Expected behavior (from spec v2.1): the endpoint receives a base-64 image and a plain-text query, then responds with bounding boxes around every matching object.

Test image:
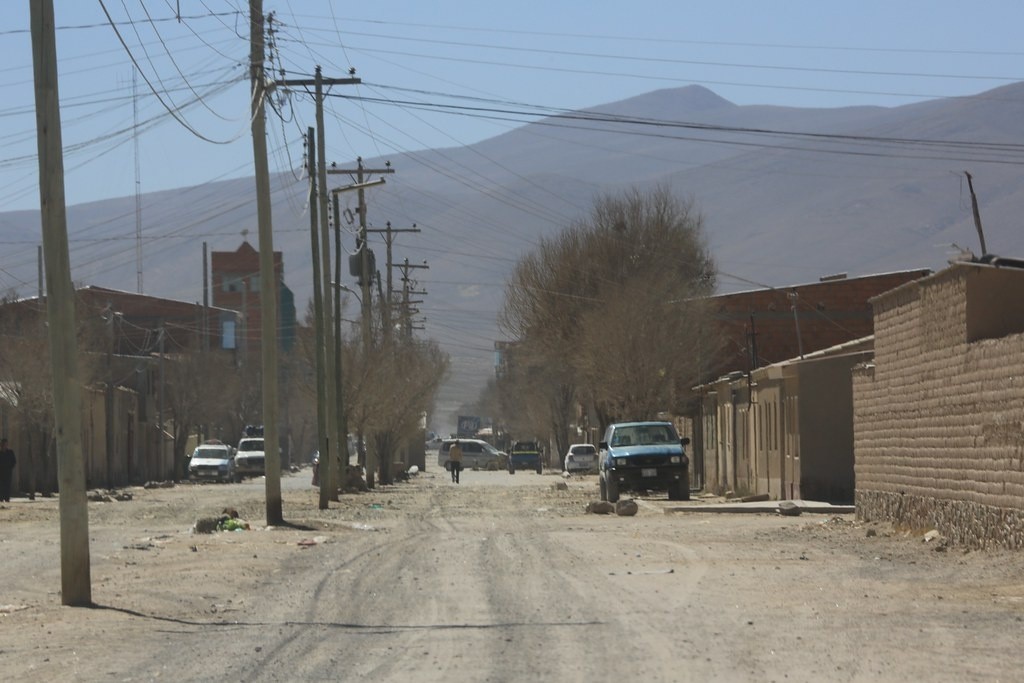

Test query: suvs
[597,422,692,501]
[509,440,544,474]
[236,438,282,478]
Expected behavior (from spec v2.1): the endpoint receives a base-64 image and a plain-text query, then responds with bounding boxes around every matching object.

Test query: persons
[449,439,462,484]
[0,439,17,502]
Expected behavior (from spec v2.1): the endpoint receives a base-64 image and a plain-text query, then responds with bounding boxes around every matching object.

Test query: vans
[438,438,510,470]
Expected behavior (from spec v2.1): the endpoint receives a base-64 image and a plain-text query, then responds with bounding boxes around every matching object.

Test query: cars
[565,444,600,472]
[188,444,235,483]
